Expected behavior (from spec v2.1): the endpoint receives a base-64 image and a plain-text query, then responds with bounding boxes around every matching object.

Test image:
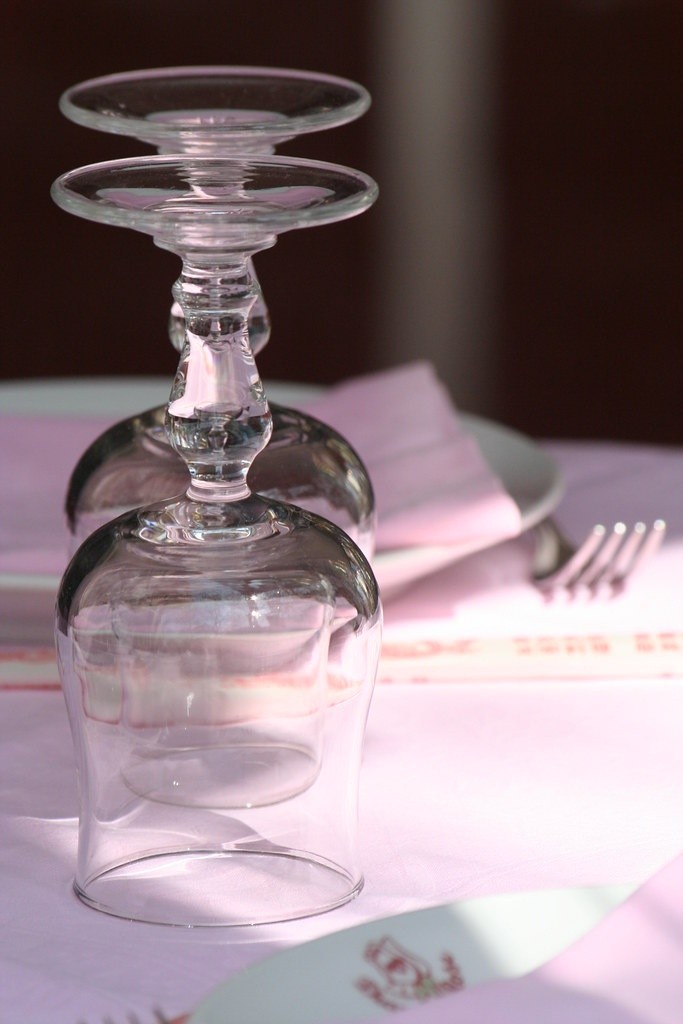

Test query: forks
[528,514,666,606]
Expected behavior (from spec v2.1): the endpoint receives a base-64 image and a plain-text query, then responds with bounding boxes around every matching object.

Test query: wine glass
[61,66,384,807]
[52,151,387,927]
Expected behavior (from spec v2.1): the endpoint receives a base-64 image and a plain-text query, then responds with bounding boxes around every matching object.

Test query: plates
[1,377,567,652]
